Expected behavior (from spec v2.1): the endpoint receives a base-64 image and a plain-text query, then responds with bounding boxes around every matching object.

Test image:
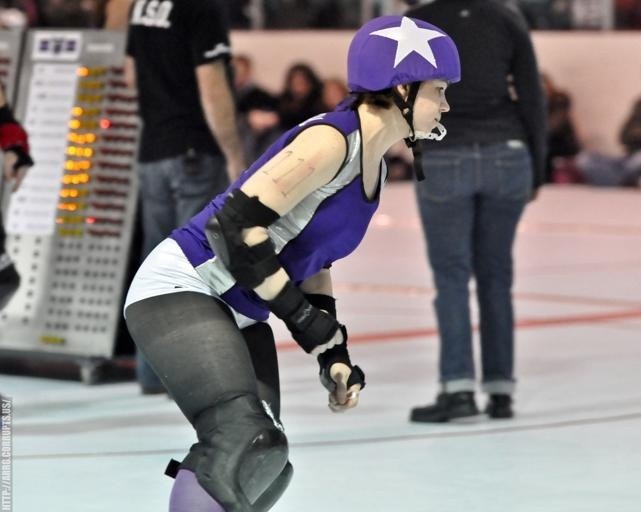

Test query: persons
[111,0,252,394]
[111,13,466,512]
[215,42,641,194]
[392,0,551,423]
[0,81,37,422]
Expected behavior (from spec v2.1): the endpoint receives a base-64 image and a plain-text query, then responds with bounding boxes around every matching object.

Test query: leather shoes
[407,390,482,424]
[484,393,517,421]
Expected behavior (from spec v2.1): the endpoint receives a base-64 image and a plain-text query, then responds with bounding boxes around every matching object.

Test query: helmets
[345,13,464,94]
